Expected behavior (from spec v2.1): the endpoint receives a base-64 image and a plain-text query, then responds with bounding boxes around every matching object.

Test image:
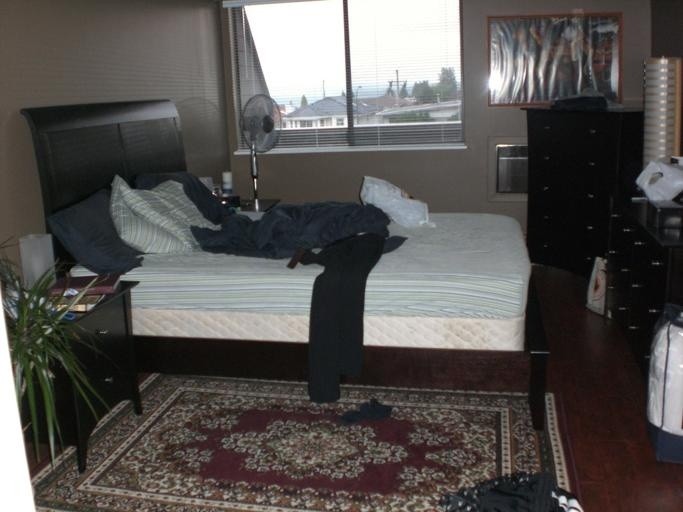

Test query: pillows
[173,172,234,224]
[110,175,191,254]
[124,186,222,248]
[48,189,142,277]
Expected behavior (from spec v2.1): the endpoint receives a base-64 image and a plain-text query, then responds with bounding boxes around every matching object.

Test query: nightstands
[238,199,280,212]
[12,281,142,471]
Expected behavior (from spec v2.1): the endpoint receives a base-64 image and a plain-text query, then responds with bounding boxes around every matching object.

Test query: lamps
[20,231,57,292]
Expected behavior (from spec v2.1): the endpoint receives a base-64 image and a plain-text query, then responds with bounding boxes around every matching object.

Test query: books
[51,274,122,293]
[36,292,104,314]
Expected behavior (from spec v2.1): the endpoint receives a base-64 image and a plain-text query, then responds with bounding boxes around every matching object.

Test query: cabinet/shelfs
[528,110,644,276]
[610,199,683,379]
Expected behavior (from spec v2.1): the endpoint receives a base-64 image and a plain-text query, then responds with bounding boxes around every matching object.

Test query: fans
[240,93,280,211]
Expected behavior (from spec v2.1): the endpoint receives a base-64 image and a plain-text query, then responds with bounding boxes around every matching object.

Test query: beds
[23,100,551,427]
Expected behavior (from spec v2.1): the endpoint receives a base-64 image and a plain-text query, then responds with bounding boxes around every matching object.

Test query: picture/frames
[487,14,621,108]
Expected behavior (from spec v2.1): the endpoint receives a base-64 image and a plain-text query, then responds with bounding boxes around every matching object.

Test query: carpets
[31,374,573,512]
